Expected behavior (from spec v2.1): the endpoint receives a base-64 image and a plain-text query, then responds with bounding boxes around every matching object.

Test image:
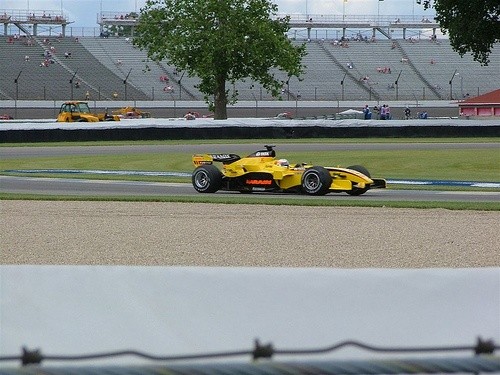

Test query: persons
[374,105,380,119]
[277,158,298,171]
[403,106,411,120]
[0,12,174,101]
[384,104,390,119]
[363,104,370,119]
[380,104,386,120]
[302,16,435,81]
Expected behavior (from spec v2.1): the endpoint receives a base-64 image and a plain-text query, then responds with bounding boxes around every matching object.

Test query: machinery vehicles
[55,101,99,122]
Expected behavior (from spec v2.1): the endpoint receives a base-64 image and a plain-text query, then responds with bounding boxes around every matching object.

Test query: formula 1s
[191,145,387,196]
